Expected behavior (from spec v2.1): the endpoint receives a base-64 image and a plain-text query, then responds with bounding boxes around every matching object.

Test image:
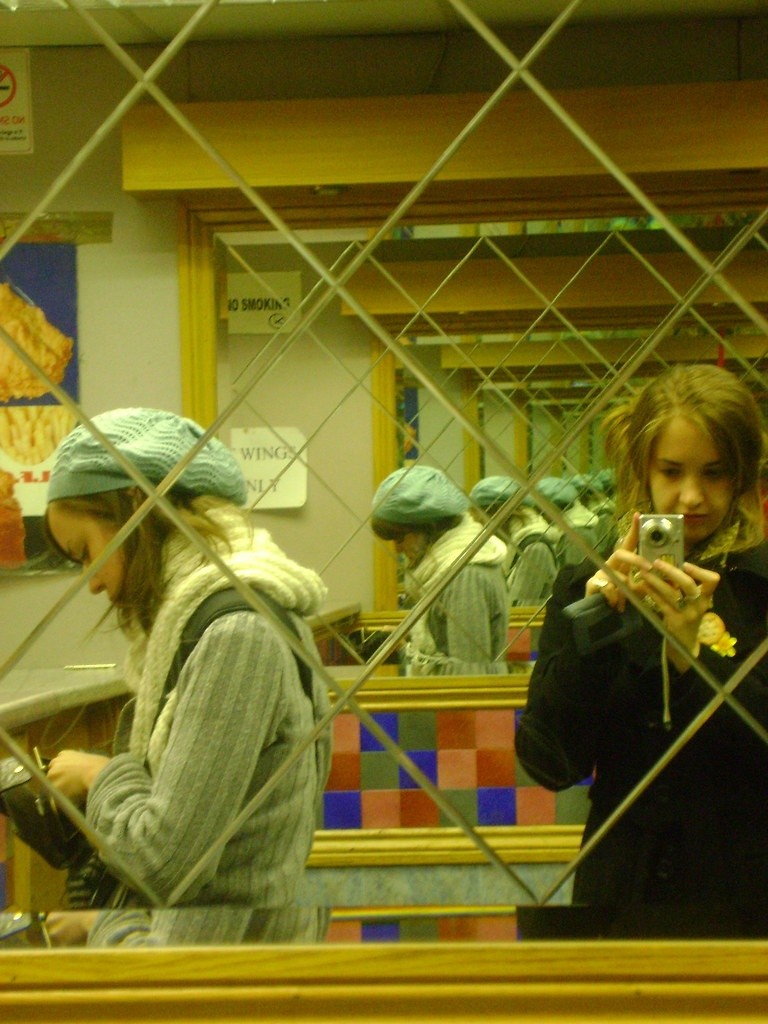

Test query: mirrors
[2,0,767,1017]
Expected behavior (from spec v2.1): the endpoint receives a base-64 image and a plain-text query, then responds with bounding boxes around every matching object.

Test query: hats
[573,471,605,501]
[596,466,621,494]
[470,475,537,509]
[540,477,581,508]
[373,463,468,523]
[46,406,250,505]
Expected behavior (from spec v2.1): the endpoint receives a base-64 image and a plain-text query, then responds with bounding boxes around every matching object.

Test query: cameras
[638,513,686,606]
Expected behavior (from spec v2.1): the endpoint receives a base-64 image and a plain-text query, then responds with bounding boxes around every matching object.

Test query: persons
[519,907,768,942]
[466,476,560,608]
[371,467,511,660]
[569,474,623,553]
[591,468,621,505]
[46,409,335,913]
[402,659,509,677]
[530,478,601,571]
[513,364,767,904]
[39,911,331,952]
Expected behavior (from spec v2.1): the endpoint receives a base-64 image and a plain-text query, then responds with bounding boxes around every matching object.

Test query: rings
[597,580,613,596]
[674,597,686,610]
[687,589,702,603]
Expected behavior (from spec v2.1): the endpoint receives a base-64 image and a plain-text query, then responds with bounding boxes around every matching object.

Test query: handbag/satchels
[1,755,88,870]
